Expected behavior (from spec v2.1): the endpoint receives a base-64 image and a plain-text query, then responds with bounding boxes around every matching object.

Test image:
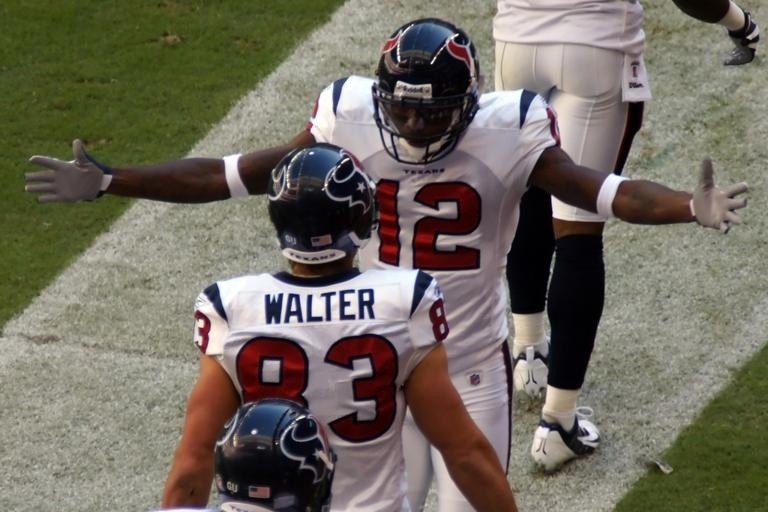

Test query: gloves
[24,139,111,204]
[724,12,759,65]
[690,157,748,232]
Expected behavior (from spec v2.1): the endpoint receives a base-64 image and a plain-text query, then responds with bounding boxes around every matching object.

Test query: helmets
[371,18,480,165]
[268,143,376,266]
[215,398,335,512]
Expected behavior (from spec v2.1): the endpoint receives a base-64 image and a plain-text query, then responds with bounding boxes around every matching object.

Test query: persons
[163,396,337,512]
[492,0,759,472]
[24,19,748,512]
[161,145,520,512]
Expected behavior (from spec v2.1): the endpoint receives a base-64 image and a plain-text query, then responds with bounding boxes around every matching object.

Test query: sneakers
[530,419,600,472]
[514,338,549,396]
